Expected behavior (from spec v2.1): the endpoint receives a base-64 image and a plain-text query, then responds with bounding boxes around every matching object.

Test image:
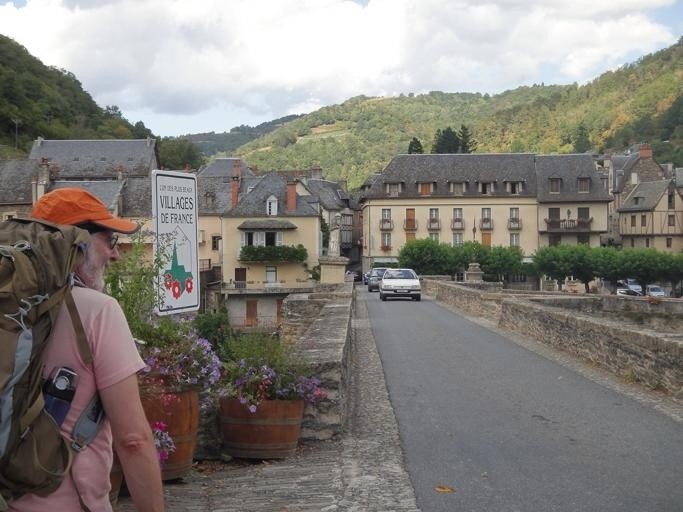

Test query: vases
[140,385,218,481]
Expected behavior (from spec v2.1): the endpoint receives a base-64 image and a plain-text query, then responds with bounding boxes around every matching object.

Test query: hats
[31,187,139,234]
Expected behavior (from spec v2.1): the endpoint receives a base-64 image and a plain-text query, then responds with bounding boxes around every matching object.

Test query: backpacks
[1,214,104,512]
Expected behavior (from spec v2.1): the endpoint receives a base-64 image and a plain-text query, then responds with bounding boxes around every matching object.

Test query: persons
[7,188,166,512]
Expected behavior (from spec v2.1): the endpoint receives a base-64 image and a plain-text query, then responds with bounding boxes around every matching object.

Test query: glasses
[107,234,117,249]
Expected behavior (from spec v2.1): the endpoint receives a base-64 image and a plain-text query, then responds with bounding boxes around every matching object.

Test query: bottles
[41,364,79,427]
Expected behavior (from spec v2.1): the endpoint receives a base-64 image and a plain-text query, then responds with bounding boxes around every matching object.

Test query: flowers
[126,318,329,461]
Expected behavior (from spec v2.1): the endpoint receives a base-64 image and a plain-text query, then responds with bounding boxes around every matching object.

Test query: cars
[364,267,421,301]
[616,279,667,297]
